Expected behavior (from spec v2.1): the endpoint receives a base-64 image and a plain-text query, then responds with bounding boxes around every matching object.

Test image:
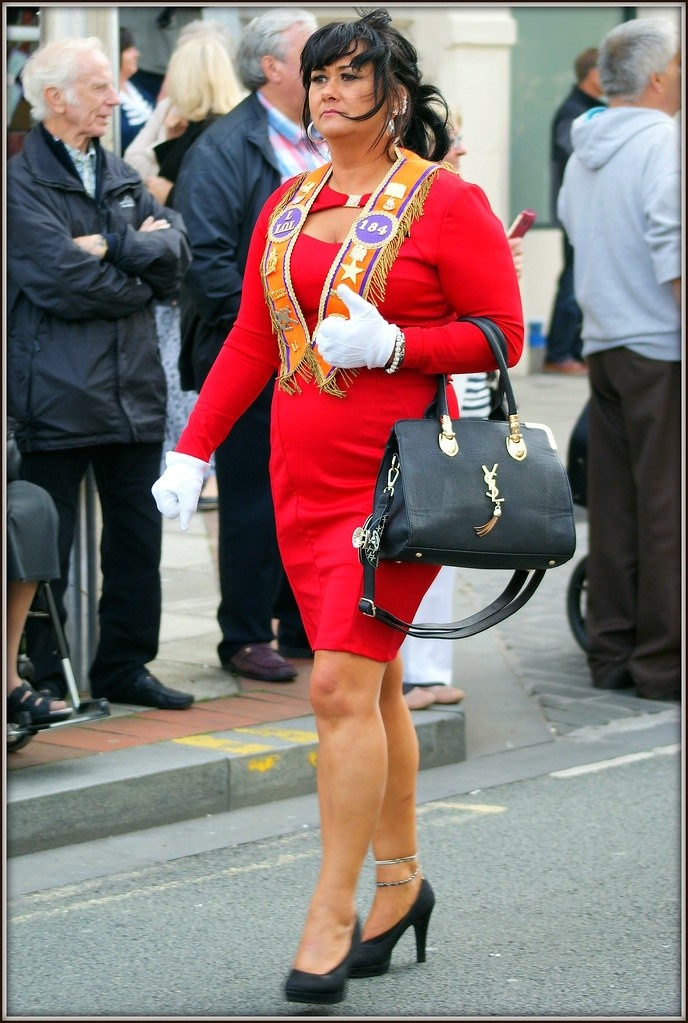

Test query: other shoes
[198,492,222,511]
[539,356,592,375]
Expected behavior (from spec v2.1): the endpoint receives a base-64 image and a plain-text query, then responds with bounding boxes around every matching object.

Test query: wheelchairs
[7,574,110,753]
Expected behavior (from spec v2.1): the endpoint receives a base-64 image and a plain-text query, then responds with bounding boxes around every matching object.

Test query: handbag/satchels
[350,317,576,639]
[567,397,588,509]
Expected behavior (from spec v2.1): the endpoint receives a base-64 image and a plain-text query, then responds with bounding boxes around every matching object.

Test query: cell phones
[505,209,536,240]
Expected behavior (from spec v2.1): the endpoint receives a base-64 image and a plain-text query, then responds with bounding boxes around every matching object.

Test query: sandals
[8,679,74,720]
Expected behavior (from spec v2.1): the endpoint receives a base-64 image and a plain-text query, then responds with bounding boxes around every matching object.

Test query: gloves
[151,451,211,531]
[316,284,395,369]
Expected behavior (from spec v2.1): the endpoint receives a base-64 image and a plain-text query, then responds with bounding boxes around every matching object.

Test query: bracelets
[385,327,405,375]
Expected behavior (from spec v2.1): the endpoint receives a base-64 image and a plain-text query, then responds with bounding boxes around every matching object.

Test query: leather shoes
[92,673,194,710]
[221,642,298,680]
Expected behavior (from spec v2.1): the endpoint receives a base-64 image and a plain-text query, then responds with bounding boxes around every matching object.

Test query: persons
[424,104,525,420]
[6,416,74,718]
[171,9,332,682]
[151,9,525,1006]
[555,17,681,700]
[6,36,193,710]
[126,20,245,509]
[119,24,157,158]
[541,47,608,378]
[397,566,465,711]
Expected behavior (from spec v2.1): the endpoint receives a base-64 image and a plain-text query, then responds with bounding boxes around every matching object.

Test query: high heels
[284,915,361,1003]
[348,878,436,977]
